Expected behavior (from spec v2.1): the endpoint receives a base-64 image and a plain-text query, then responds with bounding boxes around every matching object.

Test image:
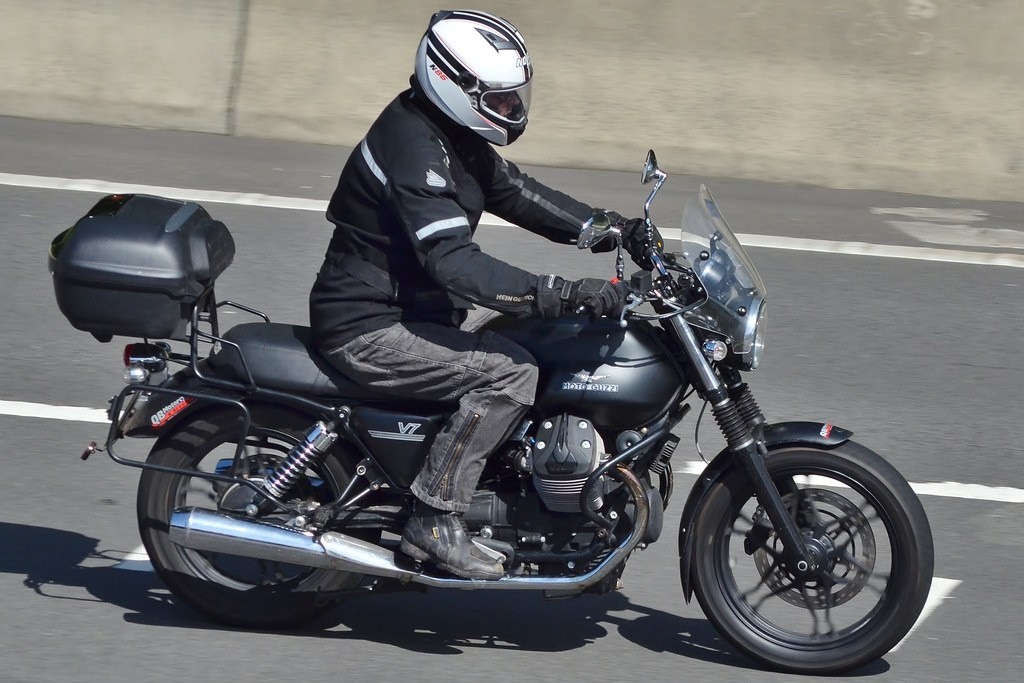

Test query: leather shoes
[399,497,504,581]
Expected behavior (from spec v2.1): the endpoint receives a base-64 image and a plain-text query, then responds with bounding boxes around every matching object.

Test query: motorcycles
[47,148,936,677]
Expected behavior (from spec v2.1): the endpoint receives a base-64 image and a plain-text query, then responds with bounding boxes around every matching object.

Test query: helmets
[414,9,533,146]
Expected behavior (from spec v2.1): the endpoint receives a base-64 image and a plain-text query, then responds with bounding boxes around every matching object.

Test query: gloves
[589,211,664,272]
[537,274,622,324]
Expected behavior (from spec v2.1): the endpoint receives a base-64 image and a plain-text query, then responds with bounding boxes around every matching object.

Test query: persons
[308,9,664,581]
